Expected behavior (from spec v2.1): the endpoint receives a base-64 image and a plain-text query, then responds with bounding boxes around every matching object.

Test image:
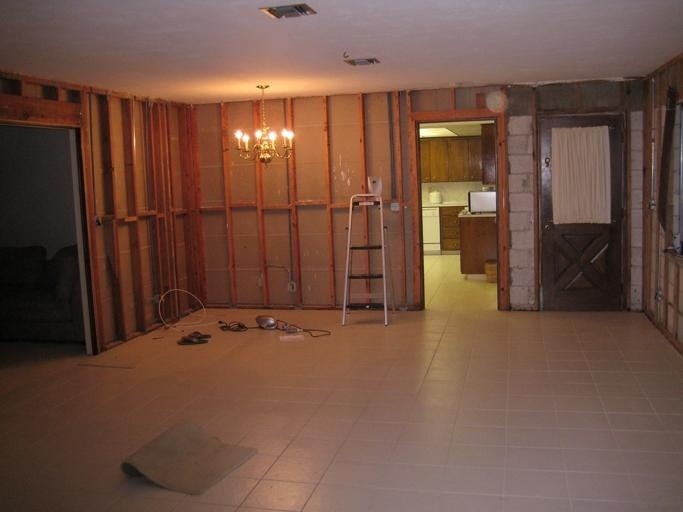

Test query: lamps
[234,84,294,167]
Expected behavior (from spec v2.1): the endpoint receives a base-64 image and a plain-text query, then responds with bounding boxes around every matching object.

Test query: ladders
[341,193,388,325]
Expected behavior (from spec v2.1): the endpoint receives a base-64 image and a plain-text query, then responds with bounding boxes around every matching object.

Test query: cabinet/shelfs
[419,135,482,183]
[438,207,467,255]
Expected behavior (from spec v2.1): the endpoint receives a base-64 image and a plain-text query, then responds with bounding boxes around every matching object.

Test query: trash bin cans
[485,260,498,282]
